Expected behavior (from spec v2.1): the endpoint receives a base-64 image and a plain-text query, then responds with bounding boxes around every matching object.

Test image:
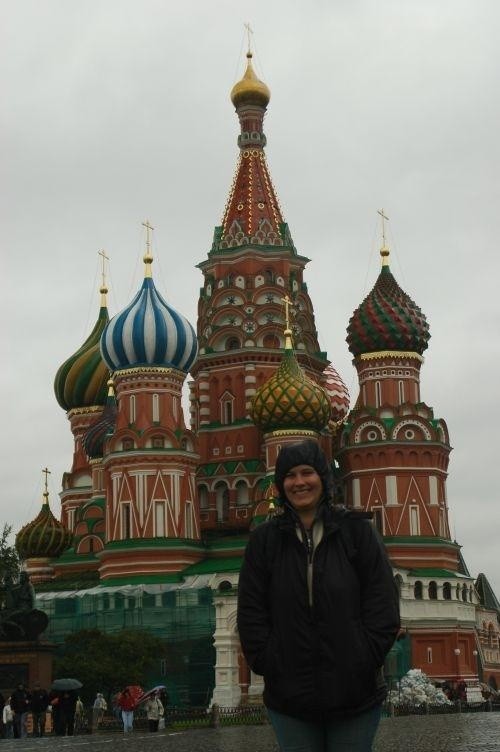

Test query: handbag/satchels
[159,717,165,730]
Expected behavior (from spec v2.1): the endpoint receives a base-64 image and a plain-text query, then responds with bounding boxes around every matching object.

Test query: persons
[1,677,109,740]
[236,435,402,751]
[117,688,136,735]
[143,692,165,732]
